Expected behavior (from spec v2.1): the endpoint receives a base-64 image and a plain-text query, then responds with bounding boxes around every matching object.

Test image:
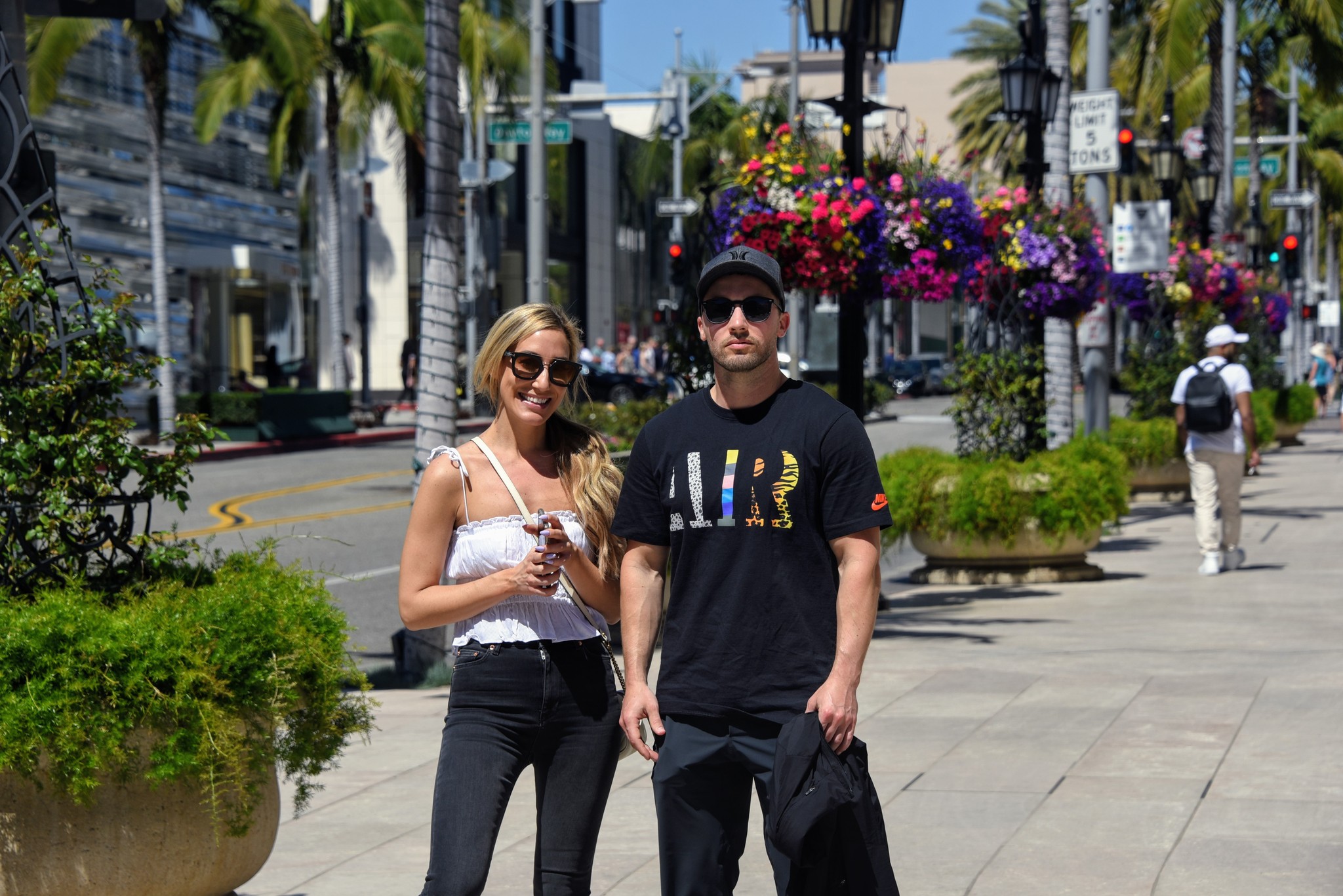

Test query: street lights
[1190,157,1221,250]
[1147,117,1184,219]
[802,0,905,424]
[996,22,1064,461]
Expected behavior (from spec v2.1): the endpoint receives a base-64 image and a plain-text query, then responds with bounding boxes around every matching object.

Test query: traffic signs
[1267,189,1320,210]
[655,196,702,217]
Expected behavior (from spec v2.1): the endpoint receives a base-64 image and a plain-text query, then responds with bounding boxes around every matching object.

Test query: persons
[1170,324,1260,575]
[399,331,420,402]
[577,336,664,381]
[399,302,625,896]
[611,246,899,896]
[1305,341,1343,430]
[334,331,352,391]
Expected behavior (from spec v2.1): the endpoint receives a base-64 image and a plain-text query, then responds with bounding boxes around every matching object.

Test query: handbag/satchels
[615,691,647,760]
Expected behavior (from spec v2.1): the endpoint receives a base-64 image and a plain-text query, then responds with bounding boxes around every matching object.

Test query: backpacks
[1184,362,1237,433]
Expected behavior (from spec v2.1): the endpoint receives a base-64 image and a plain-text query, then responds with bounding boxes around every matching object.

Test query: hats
[697,245,786,313]
[1309,341,1327,359]
[1205,324,1249,349]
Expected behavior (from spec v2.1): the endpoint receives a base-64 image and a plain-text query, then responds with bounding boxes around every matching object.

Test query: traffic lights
[1117,125,1136,176]
[1302,304,1317,319]
[1283,233,1300,279]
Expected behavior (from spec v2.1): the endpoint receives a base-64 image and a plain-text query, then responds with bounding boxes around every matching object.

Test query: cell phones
[537,508,553,590]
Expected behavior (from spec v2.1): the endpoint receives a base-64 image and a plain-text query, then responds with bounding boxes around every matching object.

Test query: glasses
[701,296,782,324]
[502,351,582,387]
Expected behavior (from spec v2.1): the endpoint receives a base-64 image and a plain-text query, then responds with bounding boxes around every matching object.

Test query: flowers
[711,108,1289,338]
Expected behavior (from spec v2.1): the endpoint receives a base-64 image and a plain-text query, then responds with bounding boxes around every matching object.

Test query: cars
[566,361,667,411]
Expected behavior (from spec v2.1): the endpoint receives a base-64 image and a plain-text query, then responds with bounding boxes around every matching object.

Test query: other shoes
[1222,548,1245,570]
[1197,556,1219,575]
